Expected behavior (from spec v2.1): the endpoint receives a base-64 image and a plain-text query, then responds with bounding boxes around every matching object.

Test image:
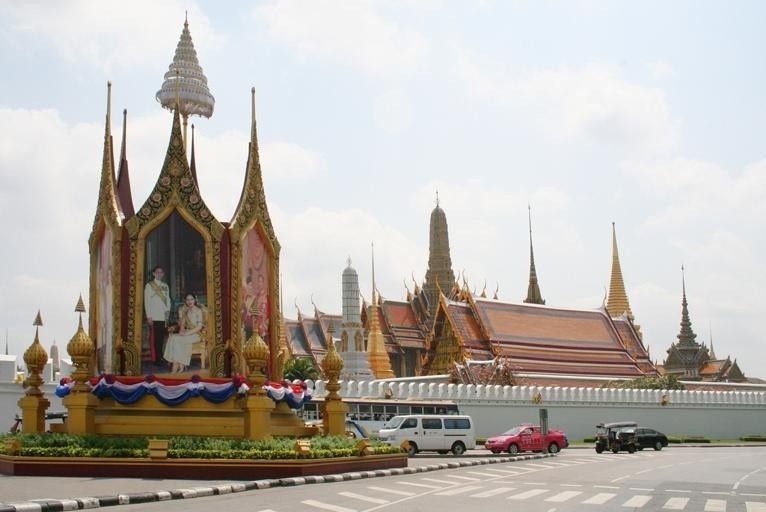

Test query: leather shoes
[157,360,188,375]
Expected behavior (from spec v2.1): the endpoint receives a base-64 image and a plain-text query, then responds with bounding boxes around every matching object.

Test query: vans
[378,415,476,457]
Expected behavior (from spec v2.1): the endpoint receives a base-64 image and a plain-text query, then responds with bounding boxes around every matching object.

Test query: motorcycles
[595,422,638,454]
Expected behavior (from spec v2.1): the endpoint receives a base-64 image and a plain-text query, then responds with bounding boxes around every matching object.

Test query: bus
[297,397,461,439]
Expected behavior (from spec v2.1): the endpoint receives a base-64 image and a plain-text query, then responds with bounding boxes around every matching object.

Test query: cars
[486,424,569,454]
[634,428,669,451]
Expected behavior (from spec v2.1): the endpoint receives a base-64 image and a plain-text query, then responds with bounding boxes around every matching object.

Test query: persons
[243,274,255,340]
[96,264,106,375]
[144,266,173,366]
[255,273,268,340]
[103,263,112,373]
[161,290,203,375]
